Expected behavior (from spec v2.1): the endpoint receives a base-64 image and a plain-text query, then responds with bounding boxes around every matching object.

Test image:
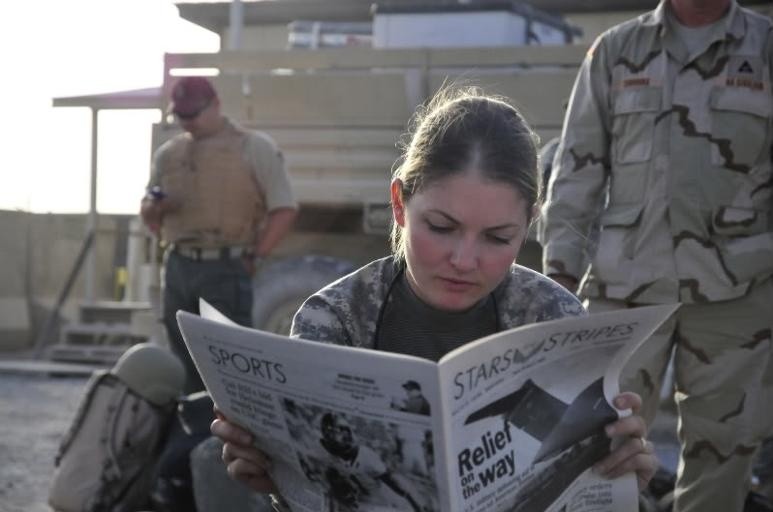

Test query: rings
[638,436,649,451]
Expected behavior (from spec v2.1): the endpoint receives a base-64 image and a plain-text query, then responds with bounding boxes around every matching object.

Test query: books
[174,297,683,512]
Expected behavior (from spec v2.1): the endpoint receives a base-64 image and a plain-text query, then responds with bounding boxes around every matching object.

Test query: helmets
[110,342,186,407]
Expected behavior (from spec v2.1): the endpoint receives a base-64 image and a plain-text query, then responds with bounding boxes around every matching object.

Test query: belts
[178,247,242,260]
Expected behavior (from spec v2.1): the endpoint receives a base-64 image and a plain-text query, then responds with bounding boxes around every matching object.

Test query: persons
[537,98,611,283]
[137,79,298,394]
[209,95,660,508]
[535,1,773,510]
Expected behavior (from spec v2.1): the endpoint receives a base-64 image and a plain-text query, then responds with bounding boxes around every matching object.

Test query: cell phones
[150,185,167,201]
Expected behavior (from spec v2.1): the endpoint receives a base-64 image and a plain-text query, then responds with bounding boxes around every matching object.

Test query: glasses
[177,101,209,118]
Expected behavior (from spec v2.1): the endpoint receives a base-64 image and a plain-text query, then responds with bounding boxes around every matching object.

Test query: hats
[165,76,213,115]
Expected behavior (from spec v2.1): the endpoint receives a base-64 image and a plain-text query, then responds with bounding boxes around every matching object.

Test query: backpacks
[47,368,177,512]
